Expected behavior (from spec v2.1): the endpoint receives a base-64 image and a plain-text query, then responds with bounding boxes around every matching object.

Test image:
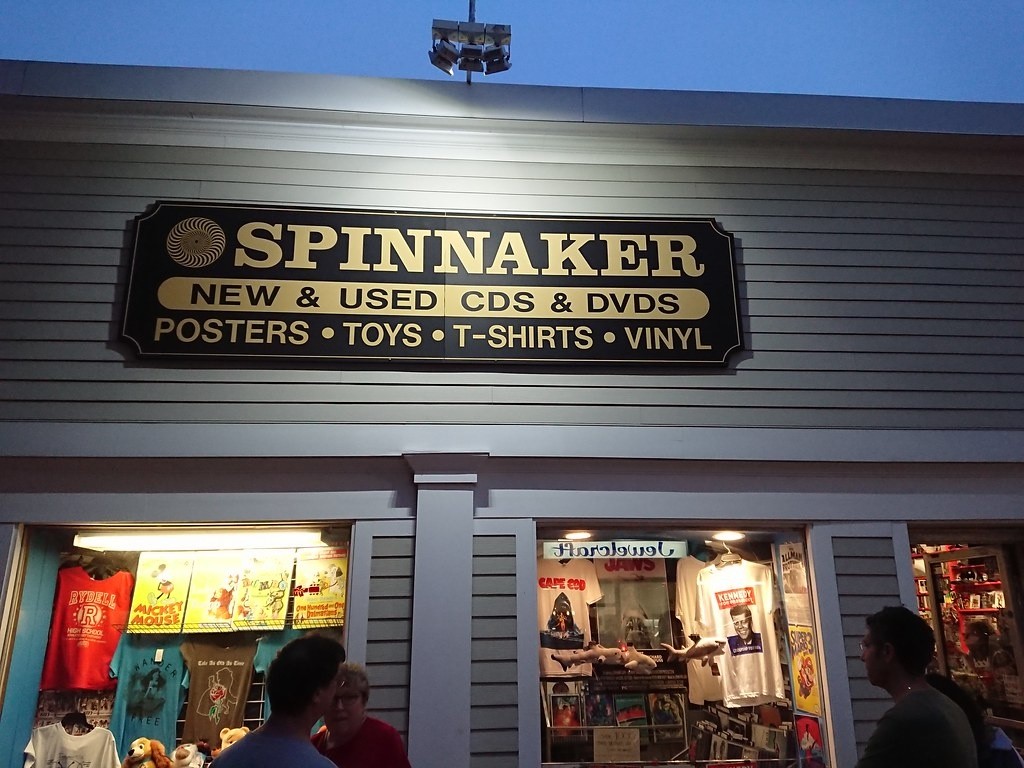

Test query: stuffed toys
[946,616,1018,702]
[550,633,726,675]
[121,724,250,768]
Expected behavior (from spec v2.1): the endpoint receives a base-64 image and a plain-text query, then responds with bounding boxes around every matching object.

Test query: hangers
[79,553,119,580]
[693,541,741,572]
[61,696,97,735]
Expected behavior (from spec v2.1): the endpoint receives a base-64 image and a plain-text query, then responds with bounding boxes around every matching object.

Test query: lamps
[74,528,332,553]
[427,0,518,84]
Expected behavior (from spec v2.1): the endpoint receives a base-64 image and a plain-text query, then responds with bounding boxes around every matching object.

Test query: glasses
[859,641,884,651]
[963,632,973,640]
[328,693,366,708]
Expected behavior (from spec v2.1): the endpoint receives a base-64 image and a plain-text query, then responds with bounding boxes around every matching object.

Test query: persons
[726,605,763,655]
[207,634,346,768]
[853,606,1024,768]
[800,722,823,764]
[310,661,413,768]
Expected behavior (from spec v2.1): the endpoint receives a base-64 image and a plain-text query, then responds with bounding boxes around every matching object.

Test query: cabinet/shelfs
[911,544,1022,701]
